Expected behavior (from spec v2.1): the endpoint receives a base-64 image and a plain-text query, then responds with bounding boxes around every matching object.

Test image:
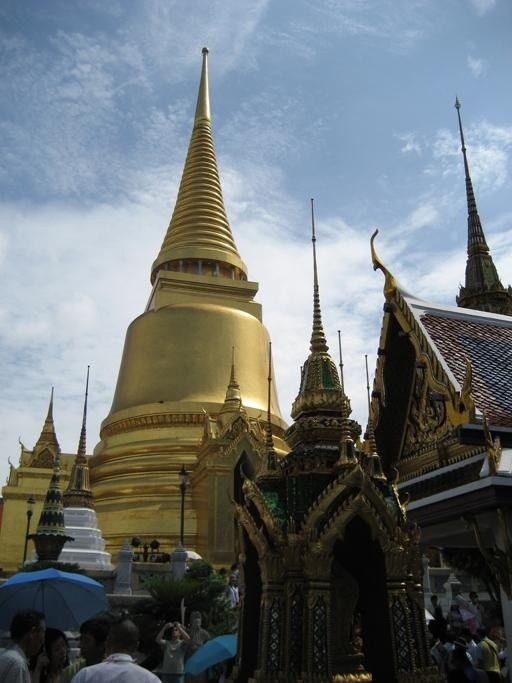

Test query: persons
[218,561,242,611]
[187,611,213,656]
[1,606,47,683]
[425,589,510,683]
[155,620,192,683]
[28,626,71,682]
[69,616,162,682]
[54,614,109,682]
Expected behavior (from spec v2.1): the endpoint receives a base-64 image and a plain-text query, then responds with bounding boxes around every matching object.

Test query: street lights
[22,492,38,562]
[177,461,191,547]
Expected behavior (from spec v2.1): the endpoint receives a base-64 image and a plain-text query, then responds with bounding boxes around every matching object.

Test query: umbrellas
[0,566,109,632]
[184,633,237,675]
